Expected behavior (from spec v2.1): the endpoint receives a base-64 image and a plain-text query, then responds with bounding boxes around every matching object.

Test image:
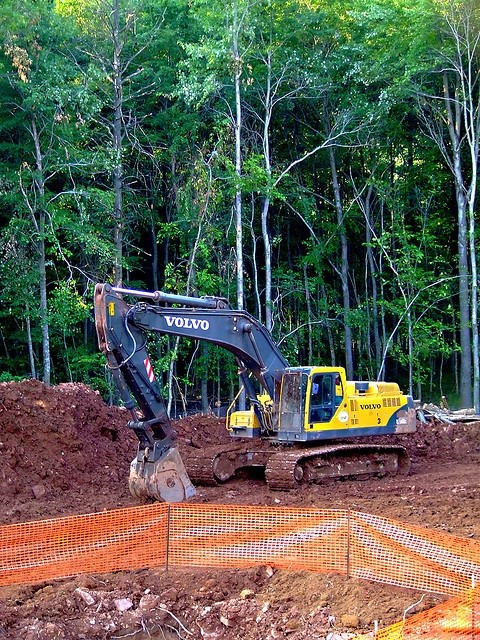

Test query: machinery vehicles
[82,277,420,507]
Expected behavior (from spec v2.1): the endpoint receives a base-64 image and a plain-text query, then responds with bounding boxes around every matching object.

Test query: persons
[312,375,327,405]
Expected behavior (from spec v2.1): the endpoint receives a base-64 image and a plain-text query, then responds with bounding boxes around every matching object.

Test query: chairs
[309,374,324,410]
